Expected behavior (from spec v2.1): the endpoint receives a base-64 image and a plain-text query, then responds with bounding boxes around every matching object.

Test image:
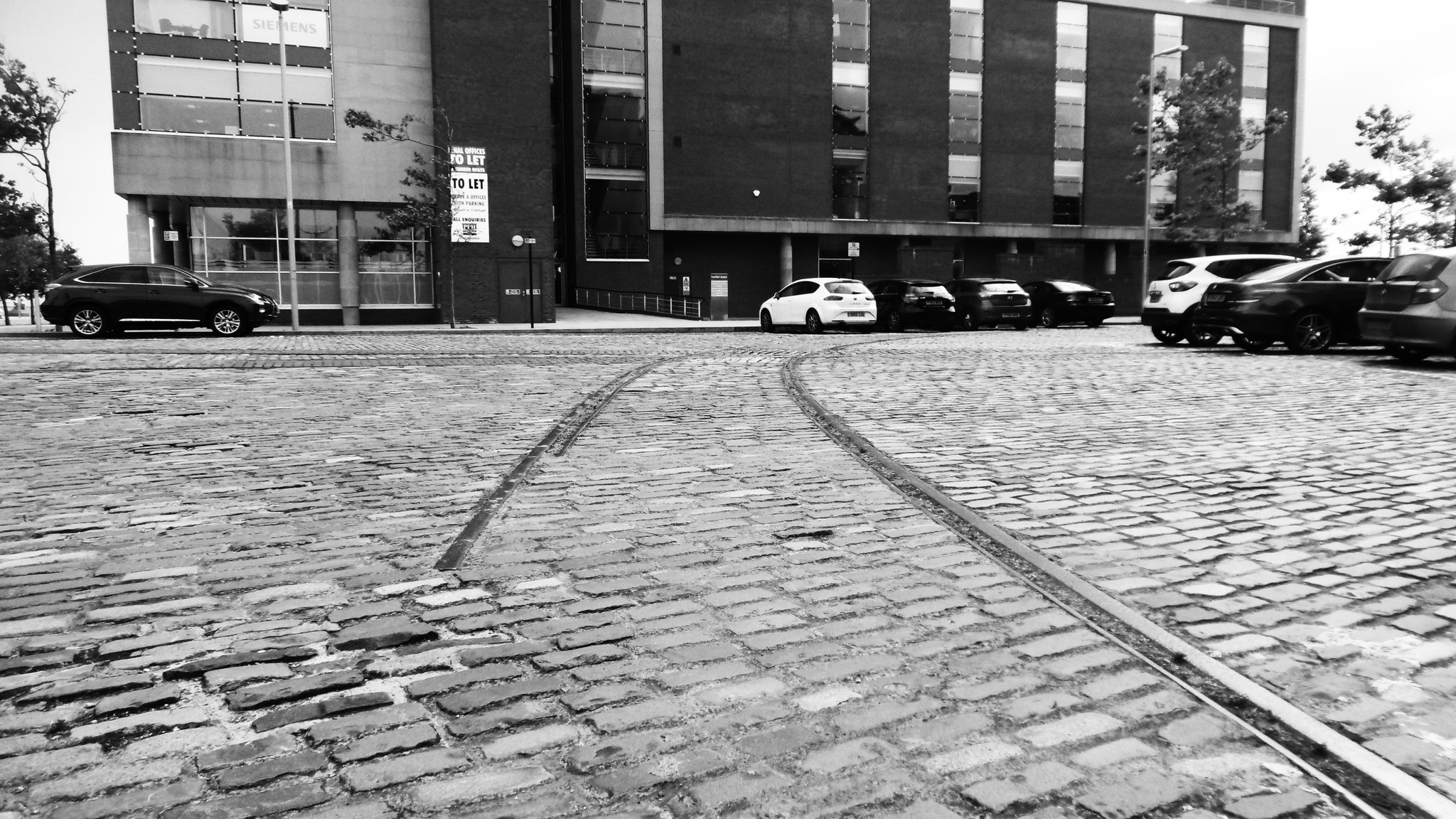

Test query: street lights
[1140,45,1189,321]
[270,0,301,330]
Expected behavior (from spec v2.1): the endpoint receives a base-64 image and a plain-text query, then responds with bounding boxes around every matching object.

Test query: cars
[1358,246,1456,371]
[1195,256,1400,354]
[759,278,877,334]
[944,277,1032,330]
[40,263,279,336]
[865,279,956,332]
[1020,279,1116,329]
[1142,254,1302,348]
